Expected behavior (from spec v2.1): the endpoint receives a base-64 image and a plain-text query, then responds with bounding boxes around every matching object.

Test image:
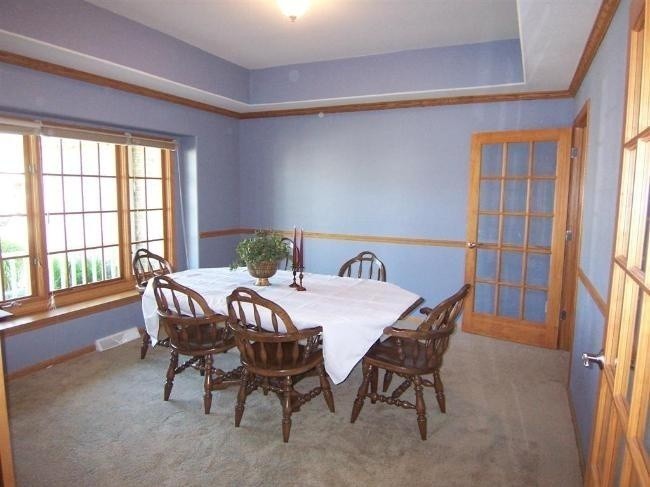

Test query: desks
[141,266,425,388]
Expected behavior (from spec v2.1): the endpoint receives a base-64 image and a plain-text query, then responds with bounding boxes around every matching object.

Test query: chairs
[133,250,205,376]
[225,288,335,442]
[279,238,299,271]
[337,251,386,282]
[350,284,472,440]
[153,276,241,415]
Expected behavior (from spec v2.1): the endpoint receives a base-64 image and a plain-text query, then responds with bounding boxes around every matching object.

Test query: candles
[293,227,297,264]
[299,228,303,267]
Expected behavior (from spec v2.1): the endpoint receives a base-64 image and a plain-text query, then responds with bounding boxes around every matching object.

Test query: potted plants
[230,230,292,285]
